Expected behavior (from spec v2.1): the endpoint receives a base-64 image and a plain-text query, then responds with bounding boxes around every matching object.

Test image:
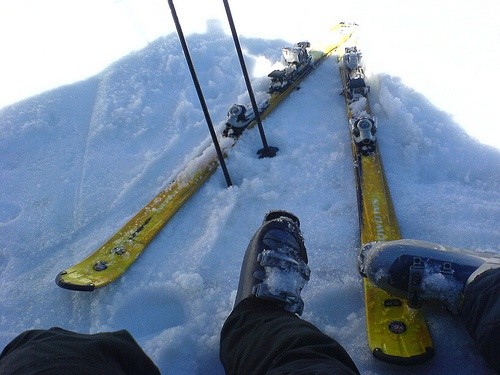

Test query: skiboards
[54,22,437,366]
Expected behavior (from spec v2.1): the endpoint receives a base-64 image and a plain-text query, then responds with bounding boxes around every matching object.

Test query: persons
[0,210,361,375]
[356,239,500,375]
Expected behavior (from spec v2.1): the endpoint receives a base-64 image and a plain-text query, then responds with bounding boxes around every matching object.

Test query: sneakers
[235,218,311,317]
[367,239,500,316]
